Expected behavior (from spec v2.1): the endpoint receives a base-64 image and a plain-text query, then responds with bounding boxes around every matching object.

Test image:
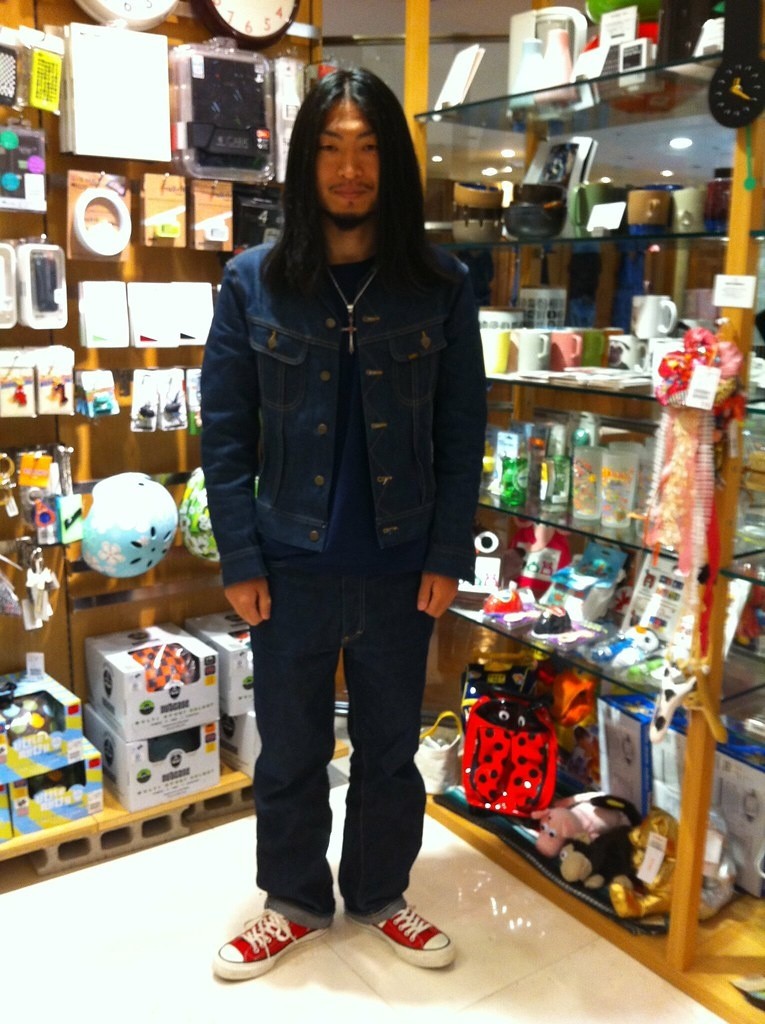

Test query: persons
[200,66,488,982]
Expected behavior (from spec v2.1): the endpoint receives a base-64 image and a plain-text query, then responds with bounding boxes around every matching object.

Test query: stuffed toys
[531,796,737,917]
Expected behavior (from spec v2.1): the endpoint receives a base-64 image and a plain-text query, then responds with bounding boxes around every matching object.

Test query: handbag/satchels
[412,710,464,795]
[462,669,559,818]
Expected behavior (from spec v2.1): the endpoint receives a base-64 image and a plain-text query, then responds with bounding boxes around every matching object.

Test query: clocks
[191,0,300,51]
[74,0,179,30]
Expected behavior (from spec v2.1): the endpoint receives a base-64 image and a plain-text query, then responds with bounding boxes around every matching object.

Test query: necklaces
[329,269,375,354]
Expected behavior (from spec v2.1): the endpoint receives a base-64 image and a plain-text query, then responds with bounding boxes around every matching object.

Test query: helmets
[180,466,219,564]
[78,471,178,580]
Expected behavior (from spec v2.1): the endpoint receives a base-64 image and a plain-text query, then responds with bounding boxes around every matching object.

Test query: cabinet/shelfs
[411,51,765,1023]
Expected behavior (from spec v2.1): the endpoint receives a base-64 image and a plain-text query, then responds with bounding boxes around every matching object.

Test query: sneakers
[212,910,332,980]
[343,907,455,968]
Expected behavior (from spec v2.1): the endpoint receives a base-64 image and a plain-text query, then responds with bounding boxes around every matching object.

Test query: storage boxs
[0,610,262,846]
[595,678,765,901]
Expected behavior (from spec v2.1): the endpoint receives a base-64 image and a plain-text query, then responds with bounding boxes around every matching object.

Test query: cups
[478,289,718,376]
[571,180,705,234]
[572,438,659,528]
